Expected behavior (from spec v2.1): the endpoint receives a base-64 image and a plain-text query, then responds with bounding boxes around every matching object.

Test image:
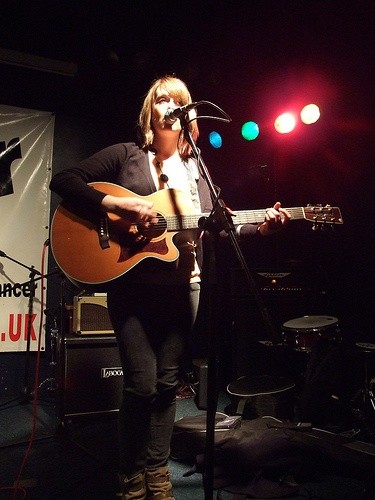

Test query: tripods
[28,317,62,402]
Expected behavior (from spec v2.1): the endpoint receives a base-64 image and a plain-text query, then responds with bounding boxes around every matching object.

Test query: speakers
[60,337,124,416]
[225,294,330,383]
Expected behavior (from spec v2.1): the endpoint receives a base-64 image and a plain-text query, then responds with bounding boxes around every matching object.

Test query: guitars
[48,176,348,293]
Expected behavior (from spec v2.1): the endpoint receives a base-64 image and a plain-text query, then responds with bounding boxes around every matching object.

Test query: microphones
[164,102,198,125]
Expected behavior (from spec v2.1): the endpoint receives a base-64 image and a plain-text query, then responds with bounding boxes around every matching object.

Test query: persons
[49,75,291,500]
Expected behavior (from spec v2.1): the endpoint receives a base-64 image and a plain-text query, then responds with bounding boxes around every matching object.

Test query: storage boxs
[52,329,124,432]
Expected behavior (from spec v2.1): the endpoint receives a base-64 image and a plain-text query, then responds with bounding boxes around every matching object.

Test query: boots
[117,466,175,500]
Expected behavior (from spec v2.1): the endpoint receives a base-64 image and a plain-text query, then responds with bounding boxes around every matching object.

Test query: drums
[284,313,346,350]
